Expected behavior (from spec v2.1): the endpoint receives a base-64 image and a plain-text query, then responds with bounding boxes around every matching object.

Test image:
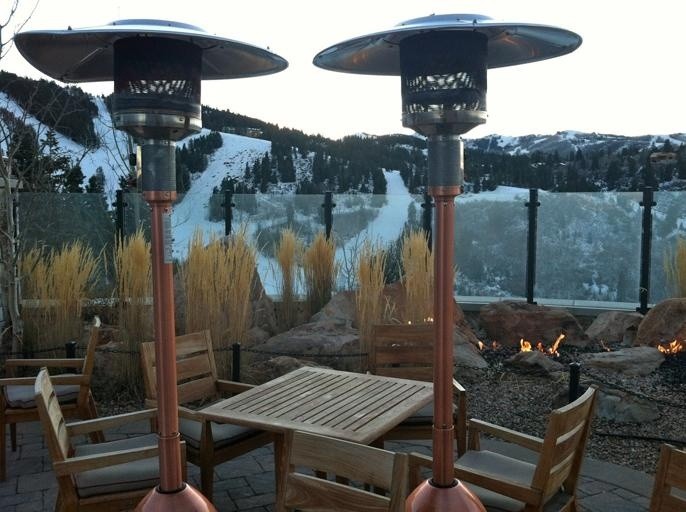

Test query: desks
[196,364,436,497]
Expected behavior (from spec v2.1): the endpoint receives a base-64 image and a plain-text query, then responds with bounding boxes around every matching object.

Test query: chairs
[30,364,187,511]
[363,322,467,496]
[136,328,274,512]
[1,313,105,481]
[644,439,686,511]
[275,432,408,511]
[404,380,600,511]
